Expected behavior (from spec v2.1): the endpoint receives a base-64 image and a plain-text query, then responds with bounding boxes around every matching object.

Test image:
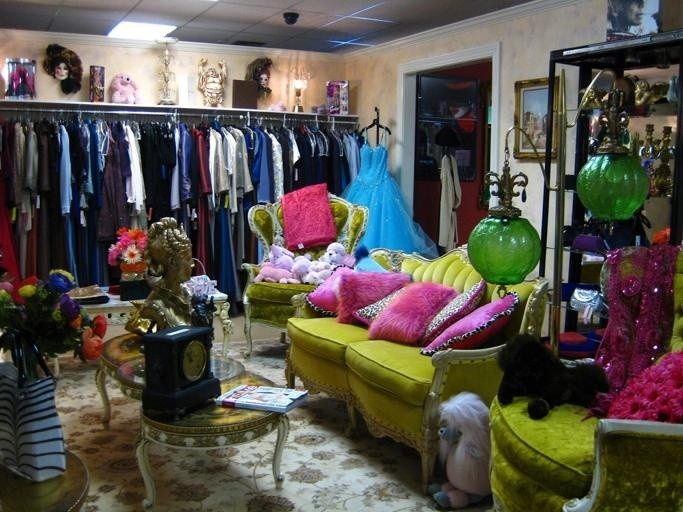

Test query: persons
[245,57,273,110]
[197,57,229,108]
[42,43,83,94]
[124,217,196,336]
[220,302,233,335]
[605,0,646,41]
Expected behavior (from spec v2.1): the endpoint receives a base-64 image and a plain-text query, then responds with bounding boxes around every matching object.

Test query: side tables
[0,448,90,512]
[47,285,236,375]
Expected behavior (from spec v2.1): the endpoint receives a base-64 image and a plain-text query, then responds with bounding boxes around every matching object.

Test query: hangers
[359,119,392,136]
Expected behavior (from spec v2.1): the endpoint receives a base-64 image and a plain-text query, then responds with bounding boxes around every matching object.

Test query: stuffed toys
[496,333,610,420]
[253,242,348,288]
[110,74,137,104]
[427,391,490,510]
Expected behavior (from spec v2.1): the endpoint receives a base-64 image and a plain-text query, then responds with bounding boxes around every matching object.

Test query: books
[216,384,310,414]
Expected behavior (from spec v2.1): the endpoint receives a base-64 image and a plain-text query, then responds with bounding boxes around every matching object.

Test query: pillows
[280,182,336,252]
[301,255,519,356]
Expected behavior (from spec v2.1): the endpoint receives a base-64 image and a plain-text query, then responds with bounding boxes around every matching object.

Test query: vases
[119,272,151,302]
[0,362,66,482]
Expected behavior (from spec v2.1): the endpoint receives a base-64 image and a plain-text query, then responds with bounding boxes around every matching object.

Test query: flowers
[108,228,149,272]
[0,268,91,387]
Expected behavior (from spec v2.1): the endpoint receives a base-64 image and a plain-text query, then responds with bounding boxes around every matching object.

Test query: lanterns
[467,160,542,298]
[575,86,649,238]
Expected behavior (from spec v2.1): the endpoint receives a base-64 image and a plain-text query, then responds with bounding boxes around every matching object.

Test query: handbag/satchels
[0,332,66,482]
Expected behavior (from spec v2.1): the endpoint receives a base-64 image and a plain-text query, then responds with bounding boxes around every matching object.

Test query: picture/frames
[513,75,559,160]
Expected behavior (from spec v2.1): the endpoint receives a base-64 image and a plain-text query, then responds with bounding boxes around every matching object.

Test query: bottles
[637,123,659,194]
[657,125,674,196]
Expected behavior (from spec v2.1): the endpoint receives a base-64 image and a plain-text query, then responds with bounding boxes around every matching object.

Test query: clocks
[143,325,222,410]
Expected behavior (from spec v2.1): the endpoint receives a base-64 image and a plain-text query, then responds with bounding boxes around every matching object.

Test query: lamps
[467,67,650,358]
[287,64,313,113]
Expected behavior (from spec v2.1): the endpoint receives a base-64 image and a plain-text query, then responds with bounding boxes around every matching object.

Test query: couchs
[284,242,549,496]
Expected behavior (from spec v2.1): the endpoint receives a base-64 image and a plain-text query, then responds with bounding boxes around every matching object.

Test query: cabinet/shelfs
[540,29,683,343]
[414,74,490,255]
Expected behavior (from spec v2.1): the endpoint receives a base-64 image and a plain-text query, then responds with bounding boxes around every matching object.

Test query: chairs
[240,192,369,359]
[487,245,683,512]
